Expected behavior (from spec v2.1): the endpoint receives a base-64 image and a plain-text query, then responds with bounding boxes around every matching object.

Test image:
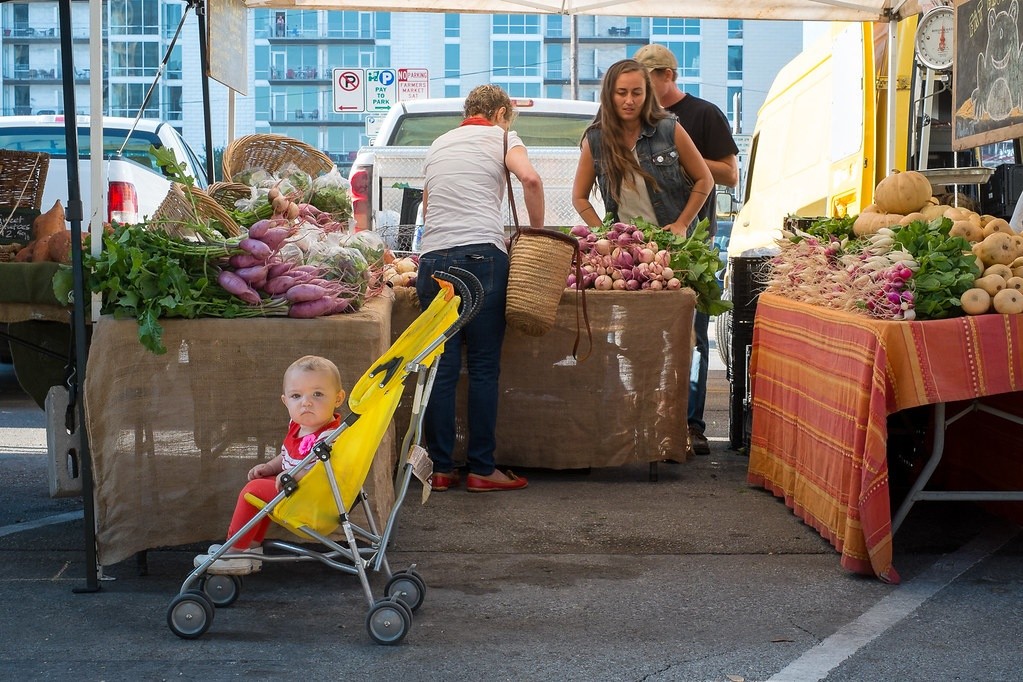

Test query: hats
[633,43,677,73]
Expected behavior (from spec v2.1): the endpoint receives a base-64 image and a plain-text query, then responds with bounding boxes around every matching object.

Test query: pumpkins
[381,249,419,286]
[850,168,1023,315]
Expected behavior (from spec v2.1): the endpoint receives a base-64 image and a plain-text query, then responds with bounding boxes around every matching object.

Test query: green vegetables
[231,164,352,222]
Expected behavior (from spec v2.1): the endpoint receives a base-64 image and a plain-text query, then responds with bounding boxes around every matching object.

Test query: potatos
[9,199,129,265]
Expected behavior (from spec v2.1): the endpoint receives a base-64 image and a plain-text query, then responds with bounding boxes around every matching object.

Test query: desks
[293,70,318,79]
[83,287,697,575]
[39,30,50,36]
[746,292,1023,586]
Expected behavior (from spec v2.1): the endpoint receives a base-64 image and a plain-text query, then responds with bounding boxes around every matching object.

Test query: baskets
[205,180,252,211]
[506,227,578,336]
[146,182,240,240]
[222,133,333,184]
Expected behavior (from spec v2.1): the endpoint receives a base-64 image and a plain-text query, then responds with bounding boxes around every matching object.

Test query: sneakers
[687,424,711,454]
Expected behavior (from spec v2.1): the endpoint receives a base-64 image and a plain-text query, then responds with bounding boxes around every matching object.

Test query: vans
[715,24,887,367]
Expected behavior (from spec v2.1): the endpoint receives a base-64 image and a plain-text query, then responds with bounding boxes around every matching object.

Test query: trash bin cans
[727,252,776,459]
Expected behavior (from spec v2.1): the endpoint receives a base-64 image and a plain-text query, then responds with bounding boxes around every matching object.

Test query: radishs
[582,237,689,290]
[753,226,919,323]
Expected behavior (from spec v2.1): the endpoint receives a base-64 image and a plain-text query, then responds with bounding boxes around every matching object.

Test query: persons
[572,60,715,237]
[418,84,543,491]
[194,355,346,575]
[632,43,738,456]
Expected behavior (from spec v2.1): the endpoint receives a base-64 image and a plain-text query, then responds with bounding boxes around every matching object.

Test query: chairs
[28,69,54,79]
[25,28,35,37]
[74,66,82,79]
[307,68,316,79]
[48,27,55,36]
[308,109,319,121]
[348,151,357,161]
[4,30,11,36]
[295,110,305,121]
[286,69,295,78]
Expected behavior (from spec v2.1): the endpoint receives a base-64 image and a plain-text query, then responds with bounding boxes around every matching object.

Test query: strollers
[166,264,485,648]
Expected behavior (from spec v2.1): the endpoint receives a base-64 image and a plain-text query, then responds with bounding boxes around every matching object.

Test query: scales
[915,6,996,187]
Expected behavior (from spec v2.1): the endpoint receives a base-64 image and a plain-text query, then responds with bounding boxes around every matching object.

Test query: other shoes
[209,544,264,572]
[432,469,460,491]
[467,470,529,491]
[194,548,254,575]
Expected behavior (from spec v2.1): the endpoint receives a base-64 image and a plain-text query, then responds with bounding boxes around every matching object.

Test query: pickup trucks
[0,114,210,237]
[347,95,610,252]
